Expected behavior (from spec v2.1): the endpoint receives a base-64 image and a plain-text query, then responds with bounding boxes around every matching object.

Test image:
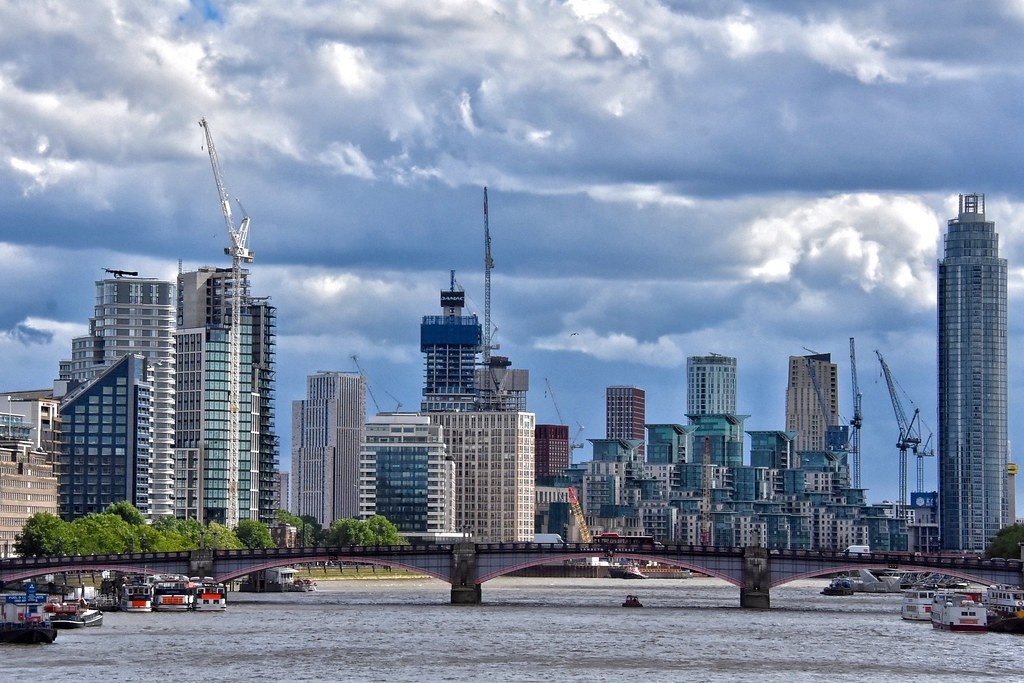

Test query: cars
[655,541,666,550]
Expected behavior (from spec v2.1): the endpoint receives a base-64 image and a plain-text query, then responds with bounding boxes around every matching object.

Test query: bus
[593,533,654,553]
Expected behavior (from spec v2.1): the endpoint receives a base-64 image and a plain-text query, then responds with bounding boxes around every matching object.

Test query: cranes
[569,487,592,542]
[916,420,934,493]
[849,335,866,488]
[198,116,255,530]
[483,185,497,411]
[873,348,919,506]
[805,360,853,454]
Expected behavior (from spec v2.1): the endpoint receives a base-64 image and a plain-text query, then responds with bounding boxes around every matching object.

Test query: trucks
[534,533,570,549]
[843,545,869,557]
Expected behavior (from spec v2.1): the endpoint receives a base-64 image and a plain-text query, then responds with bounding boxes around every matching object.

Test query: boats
[987,585,1024,613]
[50,605,104,627]
[152,574,194,612]
[191,577,227,611]
[820,577,854,596]
[932,594,987,633]
[900,589,938,621]
[608,563,647,579]
[622,595,644,608]
[1,599,59,645]
[117,571,154,613]
